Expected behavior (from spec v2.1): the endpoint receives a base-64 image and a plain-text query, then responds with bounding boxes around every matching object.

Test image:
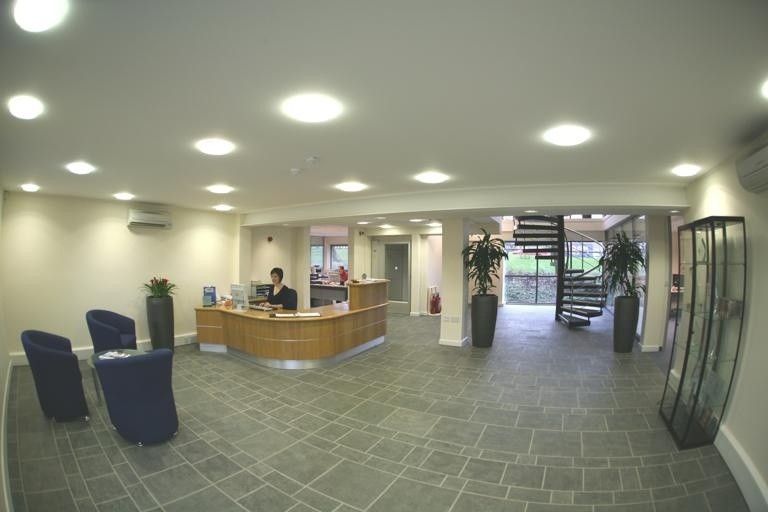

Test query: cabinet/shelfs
[311,283,348,306]
[658,216,746,451]
[251,281,274,296]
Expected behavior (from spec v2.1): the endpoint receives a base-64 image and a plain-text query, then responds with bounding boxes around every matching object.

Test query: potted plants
[461,228,509,347]
[599,230,645,353]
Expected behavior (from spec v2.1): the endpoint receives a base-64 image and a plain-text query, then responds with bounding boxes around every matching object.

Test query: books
[269,312,321,318]
[256,286,270,295]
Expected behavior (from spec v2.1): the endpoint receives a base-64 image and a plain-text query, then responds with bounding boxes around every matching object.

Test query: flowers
[143,277,177,298]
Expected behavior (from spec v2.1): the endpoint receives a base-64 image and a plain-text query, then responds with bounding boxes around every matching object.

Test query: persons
[338,265,348,286]
[259,268,288,309]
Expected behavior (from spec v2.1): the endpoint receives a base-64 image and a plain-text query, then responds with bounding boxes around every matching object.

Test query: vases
[146,296,175,354]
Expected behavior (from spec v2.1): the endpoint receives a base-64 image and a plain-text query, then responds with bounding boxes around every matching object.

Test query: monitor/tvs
[231,284,249,313]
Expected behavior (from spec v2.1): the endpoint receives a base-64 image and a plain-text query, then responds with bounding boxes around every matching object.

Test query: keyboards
[249,305,272,311]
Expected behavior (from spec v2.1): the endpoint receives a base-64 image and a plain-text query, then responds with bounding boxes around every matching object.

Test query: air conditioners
[735,144,768,194]
[126,208,173,230]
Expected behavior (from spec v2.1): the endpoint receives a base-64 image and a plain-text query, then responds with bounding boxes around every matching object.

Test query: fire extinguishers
[430,292,441,314]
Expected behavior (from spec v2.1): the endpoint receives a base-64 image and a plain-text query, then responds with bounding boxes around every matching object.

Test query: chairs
[86,309,137,353]
[21,329,89,422]
[95,348,178,447]
